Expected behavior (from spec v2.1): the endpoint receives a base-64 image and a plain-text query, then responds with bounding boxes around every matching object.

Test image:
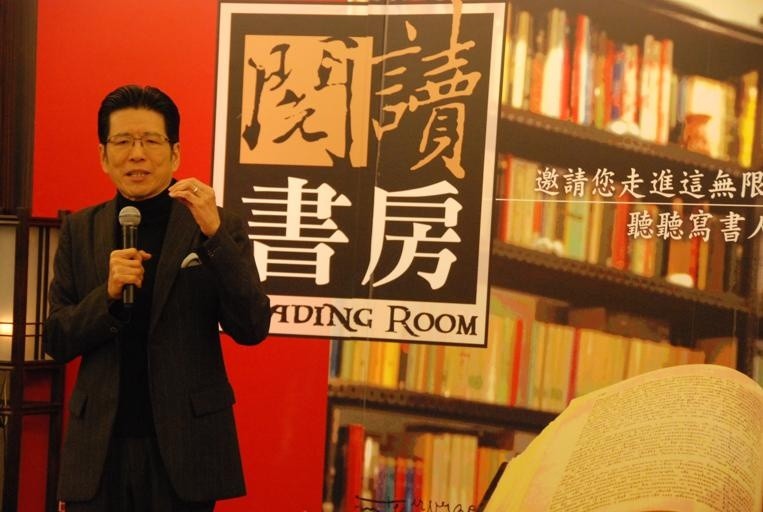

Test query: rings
[193,187,197,193]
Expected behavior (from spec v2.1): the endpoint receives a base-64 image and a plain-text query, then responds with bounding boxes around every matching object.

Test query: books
[319,10,762,512]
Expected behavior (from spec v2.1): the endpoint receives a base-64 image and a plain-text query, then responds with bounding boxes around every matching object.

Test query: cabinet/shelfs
[327,0,763,512]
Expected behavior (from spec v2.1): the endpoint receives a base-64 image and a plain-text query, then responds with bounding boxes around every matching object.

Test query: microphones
[118,205,142,309]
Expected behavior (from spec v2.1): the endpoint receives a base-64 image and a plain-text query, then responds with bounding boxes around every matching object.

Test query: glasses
[108,133,170,149]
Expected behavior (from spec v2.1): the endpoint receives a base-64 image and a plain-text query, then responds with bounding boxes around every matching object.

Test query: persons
[43,83,270,512]
